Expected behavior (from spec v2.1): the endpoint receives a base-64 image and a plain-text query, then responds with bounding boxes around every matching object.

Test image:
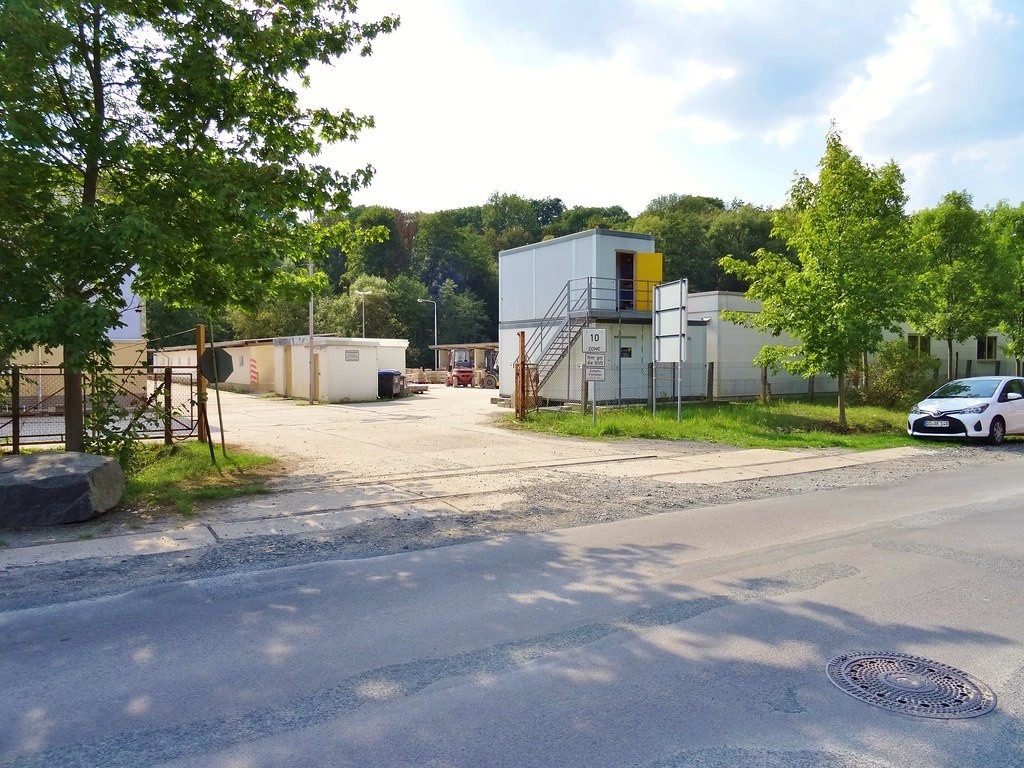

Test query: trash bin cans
[396,375,406,397]
[377,369,402,398]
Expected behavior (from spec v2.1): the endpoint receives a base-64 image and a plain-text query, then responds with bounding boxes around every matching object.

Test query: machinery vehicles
[444,348,499,390]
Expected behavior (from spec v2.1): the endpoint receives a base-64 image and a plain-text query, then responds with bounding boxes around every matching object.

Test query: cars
[908,375,1024,445]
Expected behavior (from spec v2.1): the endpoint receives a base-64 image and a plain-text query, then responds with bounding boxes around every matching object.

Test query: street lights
[417,298,439,371]
[354,290,372,338]
